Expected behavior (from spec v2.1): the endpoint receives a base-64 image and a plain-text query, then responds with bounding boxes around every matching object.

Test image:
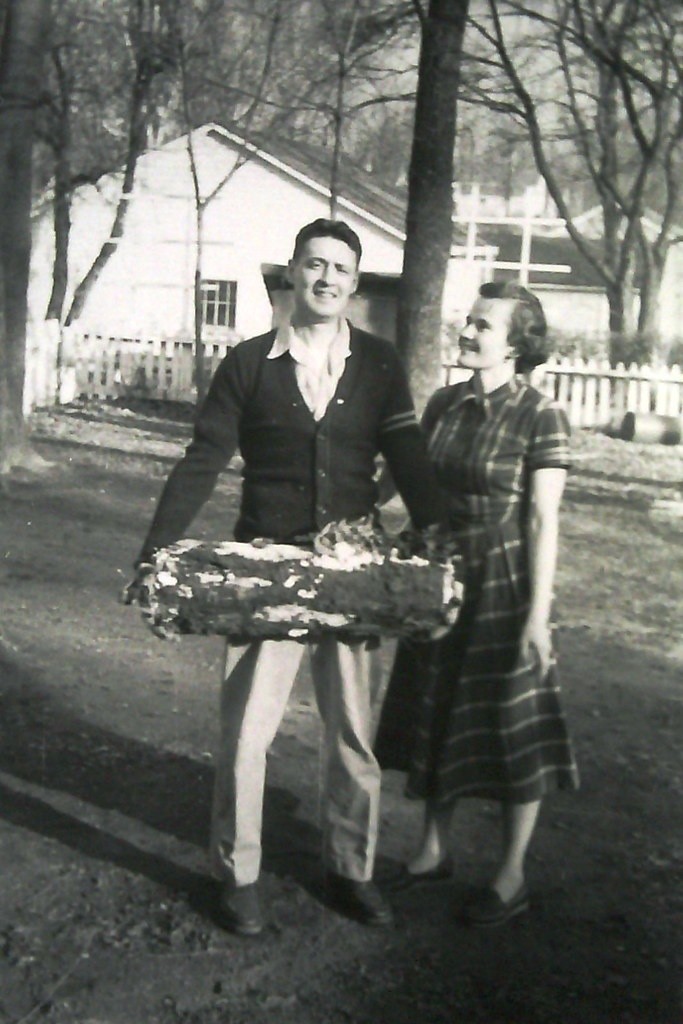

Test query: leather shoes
[325,873,392,926]
[214,870,265,937]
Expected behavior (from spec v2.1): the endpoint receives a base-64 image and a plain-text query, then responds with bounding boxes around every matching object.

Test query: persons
[122,217,464,931]
[375,281,579,928]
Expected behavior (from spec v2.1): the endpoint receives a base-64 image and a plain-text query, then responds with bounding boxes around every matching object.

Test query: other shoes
[467,879,531,931]
[390,853,456,896]
